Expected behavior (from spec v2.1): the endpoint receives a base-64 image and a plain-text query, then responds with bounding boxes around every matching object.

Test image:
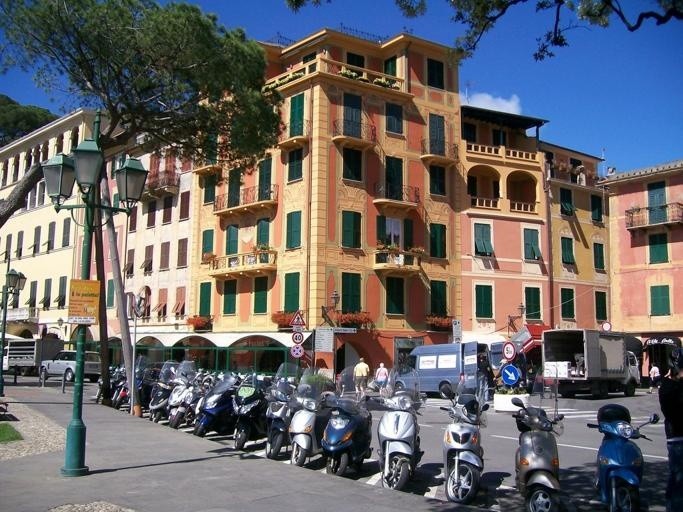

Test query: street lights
[41,107,151,477]
[0,264,26,396]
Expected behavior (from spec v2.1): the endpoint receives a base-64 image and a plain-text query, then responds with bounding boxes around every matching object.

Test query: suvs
[36,347,101,384]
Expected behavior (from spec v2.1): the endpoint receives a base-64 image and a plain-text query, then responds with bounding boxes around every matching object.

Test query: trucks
[0,336,65,379]
[538,326,641,398]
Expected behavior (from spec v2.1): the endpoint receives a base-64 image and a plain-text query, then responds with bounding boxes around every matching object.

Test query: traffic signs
[291,332,303,344]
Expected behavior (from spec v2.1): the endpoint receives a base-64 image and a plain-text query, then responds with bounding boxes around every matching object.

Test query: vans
[390,341,498,402]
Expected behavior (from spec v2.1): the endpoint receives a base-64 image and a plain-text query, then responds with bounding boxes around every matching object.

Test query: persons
[658,357,682,511]
[574,352,584,377]
[375,362,389,395]
[477,354,490,402]
[352,357,370,399]
[646,362,661,394]
[663,369,671,379]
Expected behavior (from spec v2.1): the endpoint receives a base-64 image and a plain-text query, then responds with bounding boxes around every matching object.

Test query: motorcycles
[93,354,376,478]
[508,394,564,511]
[582,400,658,512]
[437,380,487,505]
[370,361,428,492]
